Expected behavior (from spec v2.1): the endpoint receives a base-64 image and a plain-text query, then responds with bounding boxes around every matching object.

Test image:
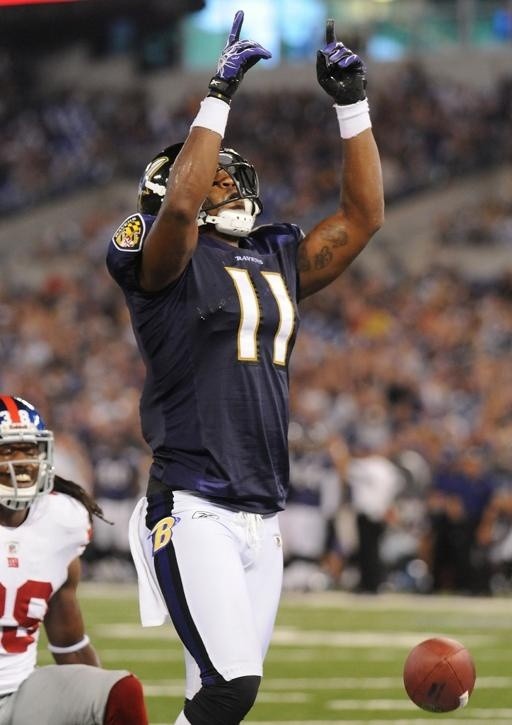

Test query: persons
[0,2,511,597]
[105,8,386,724]
[0,393,150,724]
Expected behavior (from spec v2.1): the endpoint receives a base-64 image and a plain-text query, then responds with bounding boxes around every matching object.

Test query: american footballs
[404,638,476,713]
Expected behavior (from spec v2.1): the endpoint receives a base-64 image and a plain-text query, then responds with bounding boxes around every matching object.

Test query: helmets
[1,393,62,515]
[137,139,265,239]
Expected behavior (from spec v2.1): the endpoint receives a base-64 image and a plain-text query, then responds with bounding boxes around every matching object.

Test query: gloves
[210,10,275,87]
[314,16,375,110]
